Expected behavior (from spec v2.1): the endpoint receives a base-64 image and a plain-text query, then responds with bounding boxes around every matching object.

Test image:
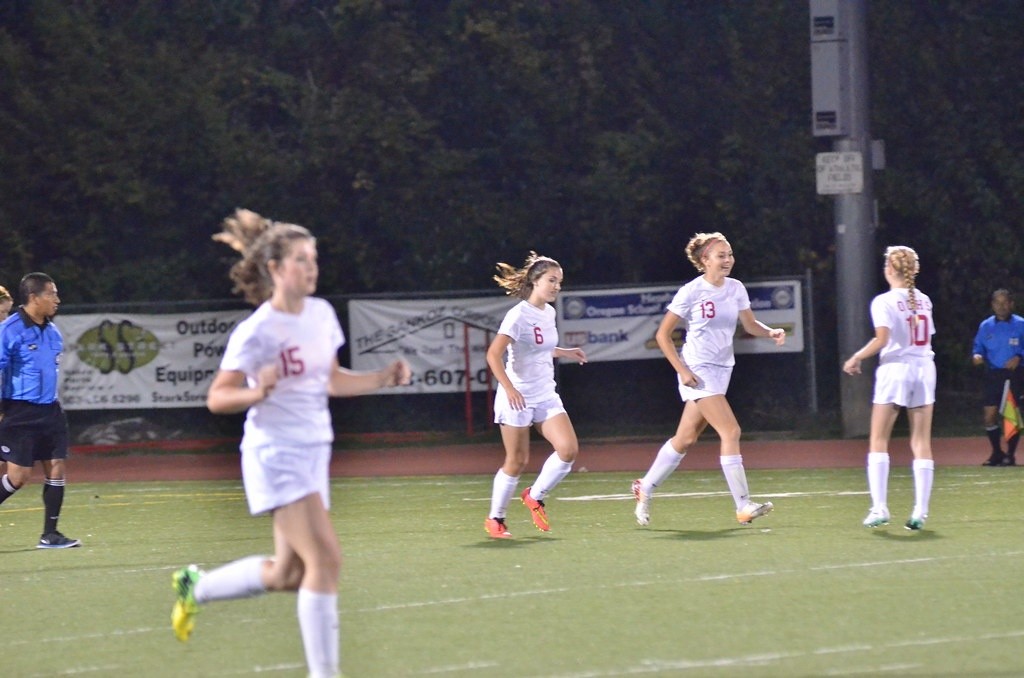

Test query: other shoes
[983,450,1006,466]
[1001,456,1015,465]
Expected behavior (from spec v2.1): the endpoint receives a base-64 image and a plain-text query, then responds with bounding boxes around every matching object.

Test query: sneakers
[171,565,207,640]
[37,530,81,548]
[521,486,551,531]
[484,517,512,538]
[737,500,774,523]
[904,518,923,530]
[863,507,890,527]
[633,478,651,525]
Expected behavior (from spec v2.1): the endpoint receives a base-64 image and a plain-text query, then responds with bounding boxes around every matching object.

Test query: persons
[483,251,587,539]
[971,287,1024,466]
[171,207,414,678]
[843,245,938,530]
[631,232,786,526]
[0,272,84,548]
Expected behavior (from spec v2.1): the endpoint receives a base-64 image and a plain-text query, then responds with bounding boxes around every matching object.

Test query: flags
[999,379,1024,442]
